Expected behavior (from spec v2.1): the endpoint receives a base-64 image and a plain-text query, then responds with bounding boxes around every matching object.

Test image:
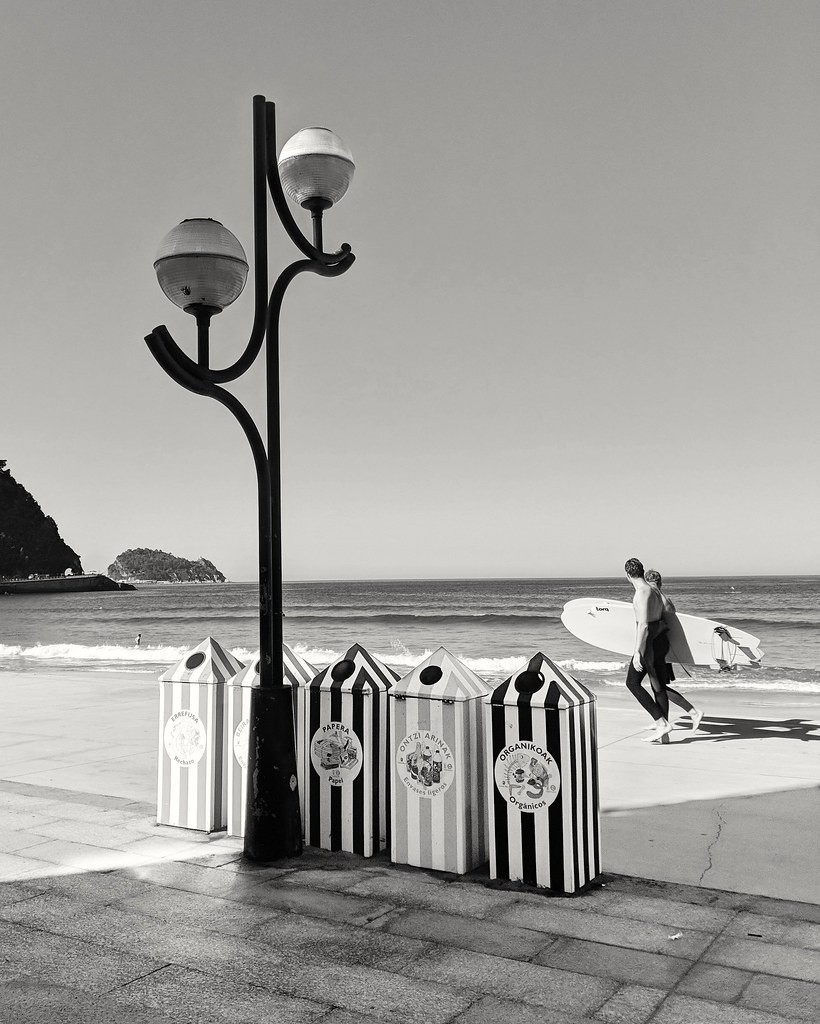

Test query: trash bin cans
[387,646,497,876]
[305,641,403,859]
[224,639,321,841]
[485,646,603,896]
[156,636,245,834]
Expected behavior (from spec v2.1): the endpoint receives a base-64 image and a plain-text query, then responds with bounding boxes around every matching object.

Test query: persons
[624,558,671,745]
[135,633,141,646]
[642,569,705,731]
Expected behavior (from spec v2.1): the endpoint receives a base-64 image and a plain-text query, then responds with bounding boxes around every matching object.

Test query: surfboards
[558,595,765,674]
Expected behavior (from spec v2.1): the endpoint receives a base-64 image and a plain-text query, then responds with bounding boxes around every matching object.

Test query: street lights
[136,90,357,870]
